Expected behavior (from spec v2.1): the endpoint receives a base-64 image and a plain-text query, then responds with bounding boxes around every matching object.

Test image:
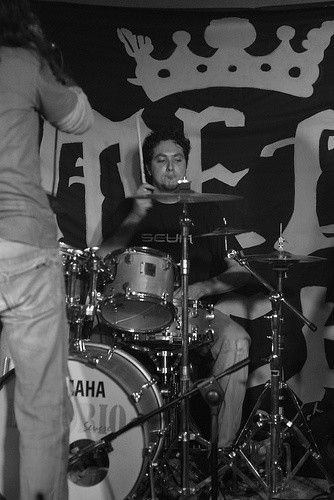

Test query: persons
[0,0,94,500]
[99,129,250,481]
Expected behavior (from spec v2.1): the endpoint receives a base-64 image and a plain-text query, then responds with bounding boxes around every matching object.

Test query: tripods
[140,204,334,500]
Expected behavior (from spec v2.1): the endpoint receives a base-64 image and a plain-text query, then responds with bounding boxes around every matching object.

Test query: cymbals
[244,251,328,265]
[127,191,245,204]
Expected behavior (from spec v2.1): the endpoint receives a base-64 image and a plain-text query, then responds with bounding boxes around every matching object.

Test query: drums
[0,342,168,500]
[96,246,178,333]
[58,240,93,325]
[138,295,215,350]
